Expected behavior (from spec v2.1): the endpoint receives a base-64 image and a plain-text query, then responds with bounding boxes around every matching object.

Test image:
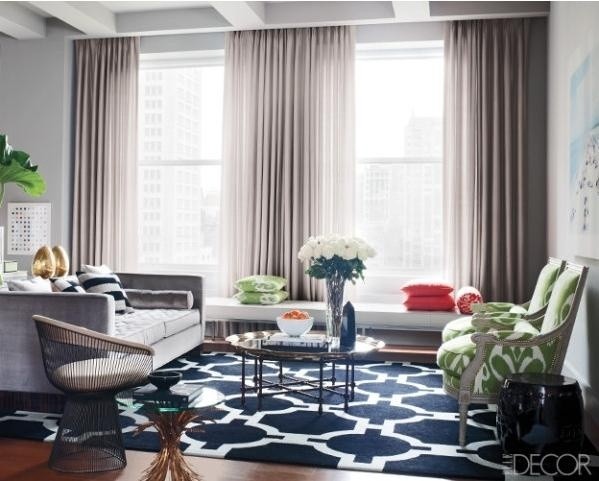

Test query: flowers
[296,230,379,337]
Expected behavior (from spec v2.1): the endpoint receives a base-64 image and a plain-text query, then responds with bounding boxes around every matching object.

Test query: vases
[323,281,345,341]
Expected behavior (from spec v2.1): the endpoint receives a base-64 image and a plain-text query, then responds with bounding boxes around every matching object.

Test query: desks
[223,329,387,418]
[114,384,226,481]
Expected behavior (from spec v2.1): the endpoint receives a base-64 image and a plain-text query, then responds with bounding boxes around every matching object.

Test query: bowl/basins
[148,371,182,391]
[276,317,314,337]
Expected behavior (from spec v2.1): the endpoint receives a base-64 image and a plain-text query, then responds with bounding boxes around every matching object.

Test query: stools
[495,371,585,462]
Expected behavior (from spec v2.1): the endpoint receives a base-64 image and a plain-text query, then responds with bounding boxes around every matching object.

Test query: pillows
[454,285,483,313]
[231,274,288,294]
[232,289,291,307]
[401,296,457,313]
[5,263,194,317]
[401,279,454,296]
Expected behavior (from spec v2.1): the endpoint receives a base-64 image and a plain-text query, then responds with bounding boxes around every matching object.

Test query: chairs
[435,261,588,451]
[25,304,159,475]
[440,256,567,345]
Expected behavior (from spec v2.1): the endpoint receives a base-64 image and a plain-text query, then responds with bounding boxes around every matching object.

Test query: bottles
[341,301,356,347]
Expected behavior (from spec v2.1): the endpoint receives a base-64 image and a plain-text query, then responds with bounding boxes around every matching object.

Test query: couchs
[0,267,206,418]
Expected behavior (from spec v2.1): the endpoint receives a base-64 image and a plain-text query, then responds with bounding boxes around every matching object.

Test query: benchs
[199,298,483,366]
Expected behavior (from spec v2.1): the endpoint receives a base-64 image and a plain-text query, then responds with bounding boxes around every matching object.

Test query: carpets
[0,343,596,481]
